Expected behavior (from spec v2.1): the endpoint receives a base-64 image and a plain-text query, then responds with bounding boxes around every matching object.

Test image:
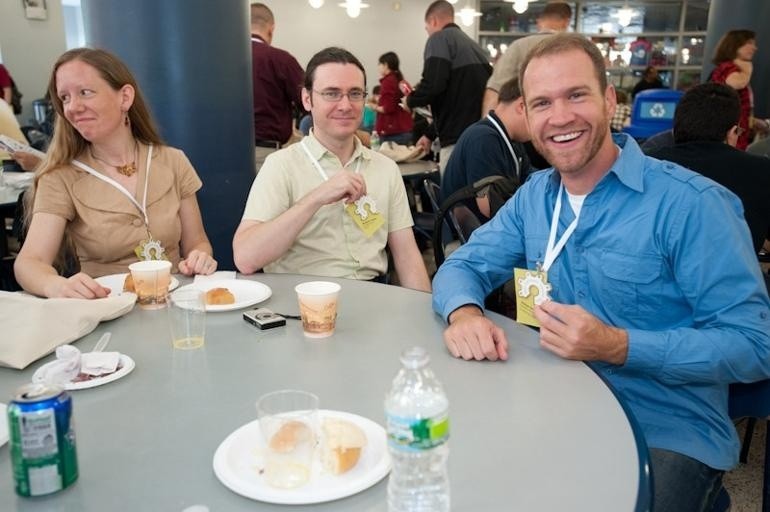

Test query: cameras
[242,306,287,331]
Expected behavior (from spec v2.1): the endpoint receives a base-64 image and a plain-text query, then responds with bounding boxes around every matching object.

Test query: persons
[645,83,770,259]
[707,29,770,151]
[365,52,412,148]
[400,0,493,184]
[0,62,56,171]
[481,3,573,122]
[631,66,663,99]
[233,47,430,294]
[431,34,770,512]
[438,77,539,224]
[13,48,218,299]
[251,3,305,163]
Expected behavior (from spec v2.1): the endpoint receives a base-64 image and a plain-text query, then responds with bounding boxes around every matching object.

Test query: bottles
[384,341,451,512]
[6,382,79,499]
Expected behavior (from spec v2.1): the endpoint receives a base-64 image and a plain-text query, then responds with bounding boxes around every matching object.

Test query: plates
[93,273,178,302]
[173,279,270,310]
[213,409,394,504]
[32,351,134,390]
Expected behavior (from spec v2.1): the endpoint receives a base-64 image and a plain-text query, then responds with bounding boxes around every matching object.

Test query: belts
[254,138,281,149]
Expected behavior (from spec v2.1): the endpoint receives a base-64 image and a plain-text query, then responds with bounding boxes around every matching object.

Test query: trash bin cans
[621,89,685,146]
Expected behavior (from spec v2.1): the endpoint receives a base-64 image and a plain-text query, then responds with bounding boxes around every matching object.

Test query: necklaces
[88,139,138,178]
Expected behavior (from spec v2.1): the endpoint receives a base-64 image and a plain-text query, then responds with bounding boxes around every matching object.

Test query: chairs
[418,178,466,255]
[431,178,520,274]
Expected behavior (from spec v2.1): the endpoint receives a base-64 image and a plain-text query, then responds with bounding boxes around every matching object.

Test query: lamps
[461,8,483,26]
[337,0,369,18]
[614,6,637,28]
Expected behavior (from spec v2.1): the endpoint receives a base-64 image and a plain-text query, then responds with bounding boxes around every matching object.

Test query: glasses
[309,89,368,101]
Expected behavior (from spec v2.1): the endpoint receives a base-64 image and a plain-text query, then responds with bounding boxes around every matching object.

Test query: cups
[130,262,172,309]
[294,282,341,337]
[164,289,203,348]
[257,390,319,486]
